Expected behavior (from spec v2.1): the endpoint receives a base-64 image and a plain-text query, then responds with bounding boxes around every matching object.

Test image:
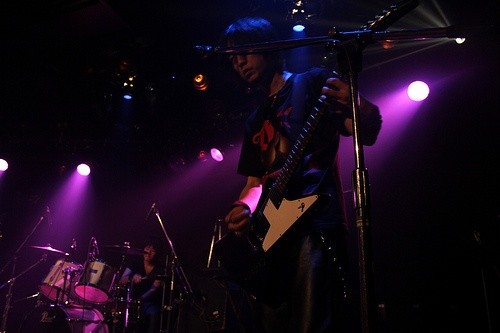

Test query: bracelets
[232,203,250,210]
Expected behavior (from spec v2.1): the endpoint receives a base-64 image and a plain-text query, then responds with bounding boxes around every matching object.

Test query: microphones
[194,45,218,59]
[144,203,156,223]
[46,206,53,224]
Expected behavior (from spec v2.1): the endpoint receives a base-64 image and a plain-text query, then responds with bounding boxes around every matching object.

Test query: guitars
[213,1,421,288]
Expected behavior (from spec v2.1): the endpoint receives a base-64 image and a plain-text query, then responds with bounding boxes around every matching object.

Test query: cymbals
[101,244,150,256]
[24,244,71,256]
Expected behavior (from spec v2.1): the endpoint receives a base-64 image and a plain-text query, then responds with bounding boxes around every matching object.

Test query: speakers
[183,268,228,333]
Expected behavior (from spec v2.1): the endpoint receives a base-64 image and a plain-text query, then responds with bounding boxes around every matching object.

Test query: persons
[119,240,168,333]
[223,15,381,333]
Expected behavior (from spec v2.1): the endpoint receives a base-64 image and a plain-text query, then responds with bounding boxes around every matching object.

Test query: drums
[110,291,146,321]
[73,260,116,305]
[18,301,109,333]
[38,259,85,306]
